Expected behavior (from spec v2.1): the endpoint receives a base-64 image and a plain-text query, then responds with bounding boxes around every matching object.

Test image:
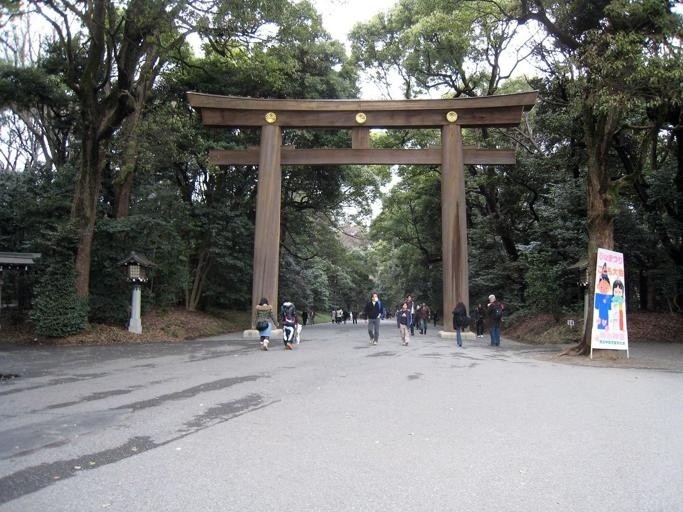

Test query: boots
[259,339,270,351]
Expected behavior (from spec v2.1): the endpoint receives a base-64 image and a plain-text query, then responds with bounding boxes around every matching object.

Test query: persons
[301,309,315,325]
[331,291,502,347]
[278,296,298,350]
[254,297,279,350]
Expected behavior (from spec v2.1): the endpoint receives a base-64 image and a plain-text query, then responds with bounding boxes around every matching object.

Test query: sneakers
[401,341,409,346]
[419,329,427,334]
[286,343,295,350]
[476,334,484,338]
[368,339,378,345]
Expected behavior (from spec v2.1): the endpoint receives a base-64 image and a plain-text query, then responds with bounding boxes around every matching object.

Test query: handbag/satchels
[255,320,269,331]
[284,314,296,326]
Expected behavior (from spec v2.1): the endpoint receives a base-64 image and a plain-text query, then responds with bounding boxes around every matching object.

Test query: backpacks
[378,300,387,320]
[490,303,502,321]
